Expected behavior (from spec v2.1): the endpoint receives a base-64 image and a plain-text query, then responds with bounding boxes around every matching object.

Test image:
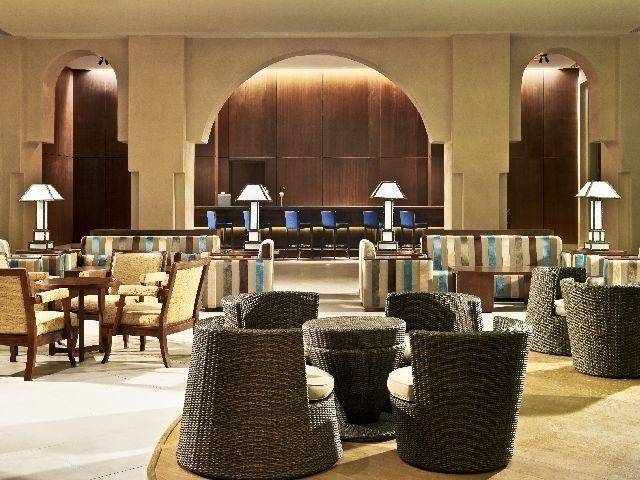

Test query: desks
[301,309,408,443]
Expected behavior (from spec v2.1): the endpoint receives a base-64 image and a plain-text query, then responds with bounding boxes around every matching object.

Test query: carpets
[145,354,640,478]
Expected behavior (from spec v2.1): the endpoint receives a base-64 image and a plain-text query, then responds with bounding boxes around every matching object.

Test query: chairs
[221,289,321,363]
[174,313,344,479]
[318,209,352,260]
[384,316,536,477]
[282,208,315,262]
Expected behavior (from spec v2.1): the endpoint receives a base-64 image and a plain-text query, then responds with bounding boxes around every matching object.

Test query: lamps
[367,174,410,252]
[572,177,623,249]
[16,183,67,250]
[235,182,275,255]
